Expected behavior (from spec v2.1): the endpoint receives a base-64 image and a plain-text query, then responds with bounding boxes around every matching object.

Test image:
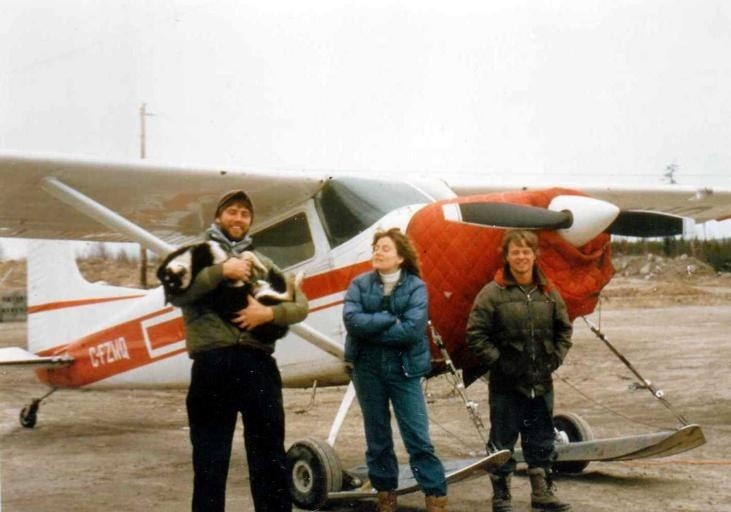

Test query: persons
[165,189,305,510]
[461,224,573,512]
[343,229,451,511]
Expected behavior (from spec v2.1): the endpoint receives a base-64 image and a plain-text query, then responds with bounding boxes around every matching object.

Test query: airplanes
[0,177,706,510]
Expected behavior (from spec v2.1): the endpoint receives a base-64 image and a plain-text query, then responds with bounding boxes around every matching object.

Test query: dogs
[156,239,305,343]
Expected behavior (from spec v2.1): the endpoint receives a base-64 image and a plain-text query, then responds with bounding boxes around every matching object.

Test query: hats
[214,186,255,226]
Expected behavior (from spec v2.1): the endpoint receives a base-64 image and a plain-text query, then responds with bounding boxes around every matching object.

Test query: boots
[525,465,571,511]
[486,461,517,512]
